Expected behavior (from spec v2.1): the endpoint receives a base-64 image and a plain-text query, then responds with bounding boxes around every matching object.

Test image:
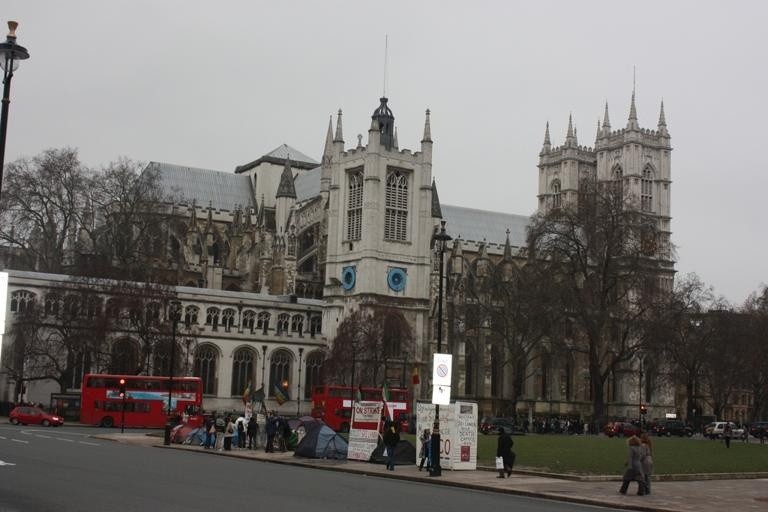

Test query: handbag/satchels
[495,456,504,469]
[623,468,636,480]
[238,421,243,431]
[229,429,233,433]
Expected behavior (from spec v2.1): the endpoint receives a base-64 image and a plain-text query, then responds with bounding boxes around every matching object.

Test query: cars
[602,421,641,437]
[9,404,64,428]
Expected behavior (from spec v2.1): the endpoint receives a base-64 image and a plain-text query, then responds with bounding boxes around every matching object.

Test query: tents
[368,440,416,466]
[288,413,349,461]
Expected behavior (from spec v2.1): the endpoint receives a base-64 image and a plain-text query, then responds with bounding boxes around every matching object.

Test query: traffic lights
[640,405,645,413]
[644,409,647,415]
[118,377,126,398]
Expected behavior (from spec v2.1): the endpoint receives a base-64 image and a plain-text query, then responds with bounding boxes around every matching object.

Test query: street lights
[163,299,183,446]
[637,350,645,423]
[260,345,267,390]
[184,338,190,375]
[426,219,453,477]
[0,20,30,198]
[295,348,304,417]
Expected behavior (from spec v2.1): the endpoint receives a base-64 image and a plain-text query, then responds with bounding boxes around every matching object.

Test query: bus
[310,382,410,433]
[78,371,204,431]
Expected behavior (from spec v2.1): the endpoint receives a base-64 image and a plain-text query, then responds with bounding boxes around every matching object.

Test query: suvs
[748,421,767,438]
[480,415,515,434]
[656,420,695,438]
[703,422,747,439]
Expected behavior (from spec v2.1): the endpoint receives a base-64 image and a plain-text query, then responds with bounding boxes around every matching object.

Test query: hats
[628,436,641,446]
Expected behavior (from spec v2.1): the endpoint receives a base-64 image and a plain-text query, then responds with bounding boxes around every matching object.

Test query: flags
[272,384,290,407]
[242,379,252,404]
[381,382,392,402]
[354,383,364,402]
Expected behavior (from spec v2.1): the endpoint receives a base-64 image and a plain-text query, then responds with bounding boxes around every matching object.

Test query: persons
[383,422,399,470]
[166,404,293,453]
[630,417,657,432]
[496,429,514,479]
[418,428,433,472]
[521,413,585,436]
[640,431,653,494]
[20,398,45,411]
[616,434,646,497]
[721,419,767,449]
